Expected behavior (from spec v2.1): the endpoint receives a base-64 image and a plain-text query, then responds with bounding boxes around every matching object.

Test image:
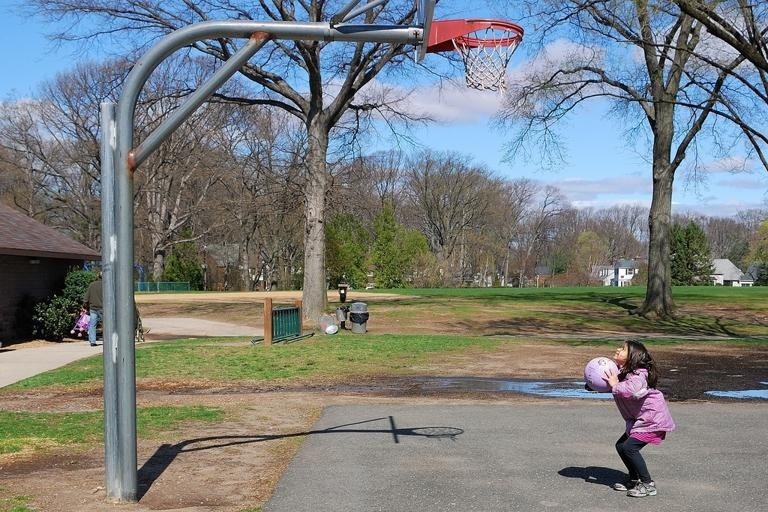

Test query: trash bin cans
[350,302,369,334]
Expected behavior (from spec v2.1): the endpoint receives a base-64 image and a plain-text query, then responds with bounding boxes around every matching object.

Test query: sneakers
[614,476,639,491]
[626,479,657,498]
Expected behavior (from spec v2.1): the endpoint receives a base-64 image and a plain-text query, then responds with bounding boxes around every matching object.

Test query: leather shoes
[90,342,98,347]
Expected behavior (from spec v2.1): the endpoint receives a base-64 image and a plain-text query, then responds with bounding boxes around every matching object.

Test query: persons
[82,272,105,348]
[585,338,677,498]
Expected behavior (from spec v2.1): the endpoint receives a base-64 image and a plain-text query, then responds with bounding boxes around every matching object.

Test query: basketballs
[585,357,619,390]
